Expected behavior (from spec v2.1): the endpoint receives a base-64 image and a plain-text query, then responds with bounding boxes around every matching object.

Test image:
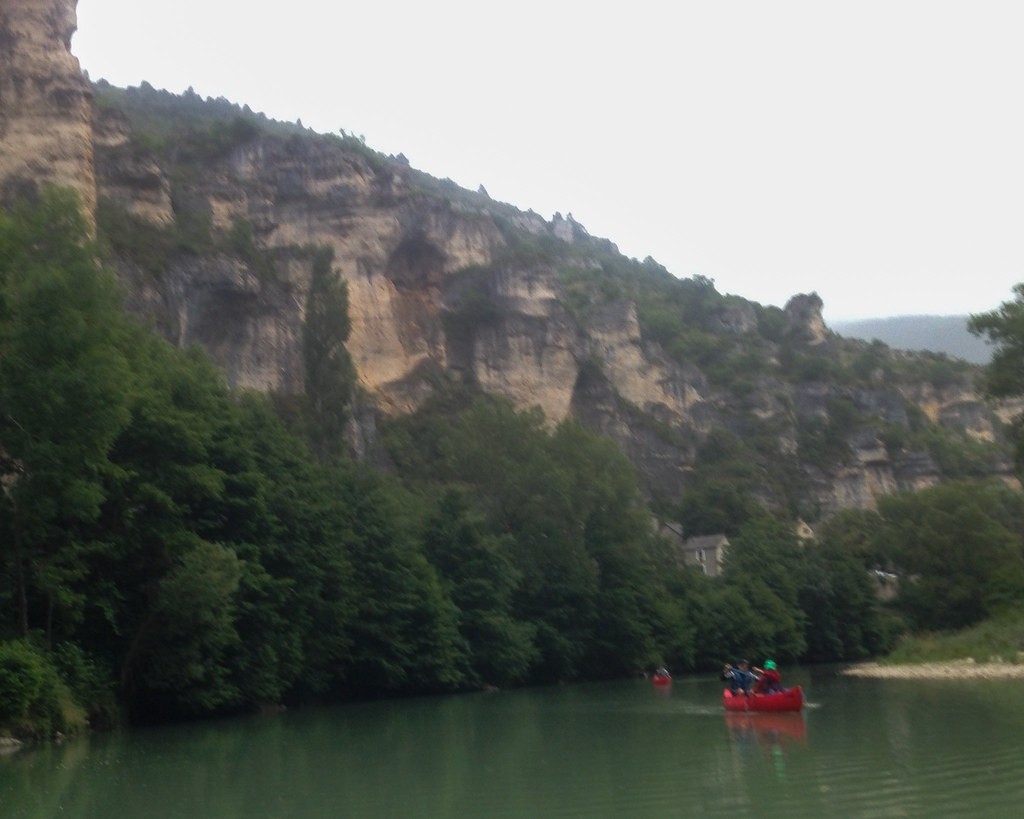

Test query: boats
[721,685,803,714]
[650,673,673,685]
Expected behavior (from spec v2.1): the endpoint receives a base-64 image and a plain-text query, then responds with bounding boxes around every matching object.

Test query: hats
[764,660,776,670]
[739,659,749,665]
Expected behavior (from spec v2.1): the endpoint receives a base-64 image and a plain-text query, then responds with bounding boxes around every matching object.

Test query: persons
[723,657,785,697]
[656,666,671,679]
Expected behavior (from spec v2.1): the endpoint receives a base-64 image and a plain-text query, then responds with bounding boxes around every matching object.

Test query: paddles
[728,665,757,680]
